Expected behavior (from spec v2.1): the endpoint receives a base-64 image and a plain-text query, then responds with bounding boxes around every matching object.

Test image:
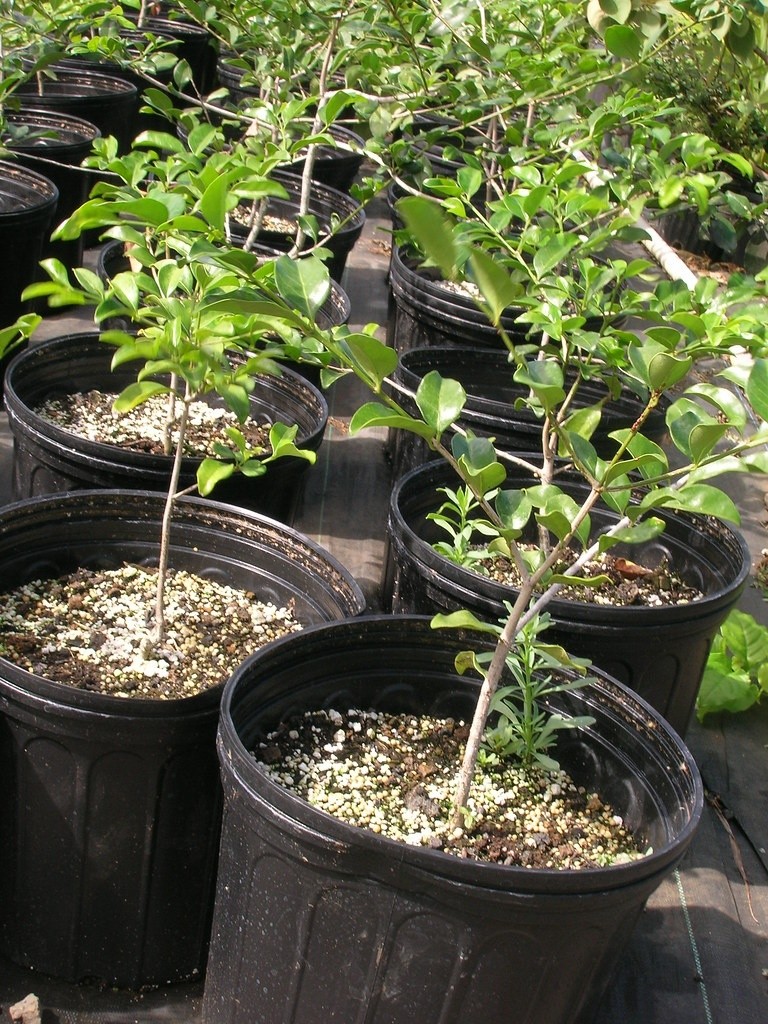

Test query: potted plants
[0,0,768,1024]
